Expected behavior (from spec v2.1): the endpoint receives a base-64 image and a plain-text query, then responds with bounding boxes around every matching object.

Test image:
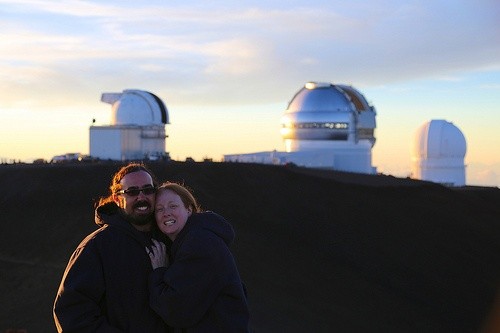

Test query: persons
[145,181,250,333]
[52,162,174,333]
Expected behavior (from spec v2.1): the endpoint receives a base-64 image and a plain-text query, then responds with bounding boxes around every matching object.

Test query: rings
[147,251,151,255]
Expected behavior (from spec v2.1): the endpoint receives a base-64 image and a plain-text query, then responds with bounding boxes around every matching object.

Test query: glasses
[116,184,155,197]
